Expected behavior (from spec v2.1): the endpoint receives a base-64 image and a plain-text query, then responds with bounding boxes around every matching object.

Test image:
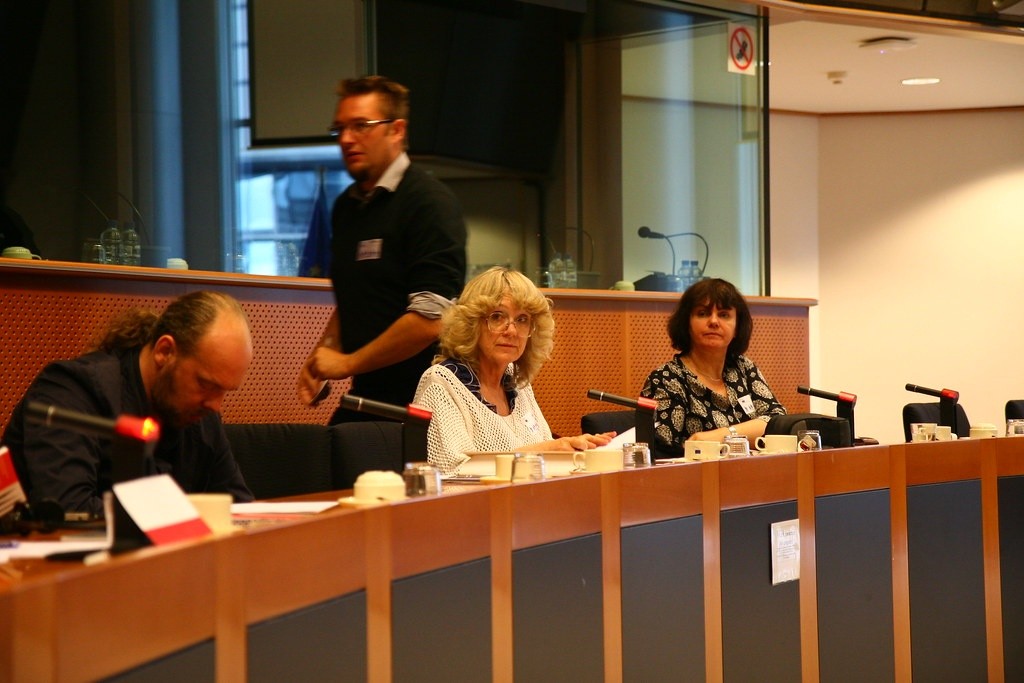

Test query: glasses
[326,119,392,136]
[480,312,533,333]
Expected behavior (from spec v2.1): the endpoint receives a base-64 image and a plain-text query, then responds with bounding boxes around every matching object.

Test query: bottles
[547,253,577,289]
[679,260,702,292]
[100,220,141,266]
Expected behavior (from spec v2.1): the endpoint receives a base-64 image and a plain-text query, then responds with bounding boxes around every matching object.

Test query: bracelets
[727,426,738,437]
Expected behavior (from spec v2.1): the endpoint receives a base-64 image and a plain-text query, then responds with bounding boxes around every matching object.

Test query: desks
[0,437,1024,683]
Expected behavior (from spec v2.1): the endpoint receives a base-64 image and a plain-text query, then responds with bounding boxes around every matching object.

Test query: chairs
[328,421,425,489]
[580,410,636,444]
[903,402,970,443]
[223,421,332,507]
[1004,400,1024,422]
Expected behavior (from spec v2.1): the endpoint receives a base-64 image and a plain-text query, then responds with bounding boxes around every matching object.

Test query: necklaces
[685,356,723,381]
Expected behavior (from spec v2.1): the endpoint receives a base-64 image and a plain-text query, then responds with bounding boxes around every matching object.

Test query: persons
[298,77,468,423]
[639,279,788,459]
[410,266,617,471]
[4,289,253,512]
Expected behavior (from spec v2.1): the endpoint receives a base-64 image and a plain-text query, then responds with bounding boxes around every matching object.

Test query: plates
[481,476,553,485]
[338,496,410,510]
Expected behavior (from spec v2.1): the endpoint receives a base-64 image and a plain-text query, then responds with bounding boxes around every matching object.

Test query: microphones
[906,384,959,401]
[74,187,151,245]
[535,224,595,270]
[638,227,709,275]
[798,385,857,402]
[25,403,159,446]
[587,389,660,411]
[340,395,433,427]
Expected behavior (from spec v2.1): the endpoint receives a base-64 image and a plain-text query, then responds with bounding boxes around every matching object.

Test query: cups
[685,440,730,464]
[573,443,651,472]
[403,461,443,498]
[755,435,798,454]
[354,470,405,500]
[722,436,750,458]
[608,279,635,291]
[910,423,952,443]
[1,247,41,260]
[969,418,1024,440]
[797,430,822,453]
[167,258,189,269]
[188,493,233,533]
[496,454,545,484]
